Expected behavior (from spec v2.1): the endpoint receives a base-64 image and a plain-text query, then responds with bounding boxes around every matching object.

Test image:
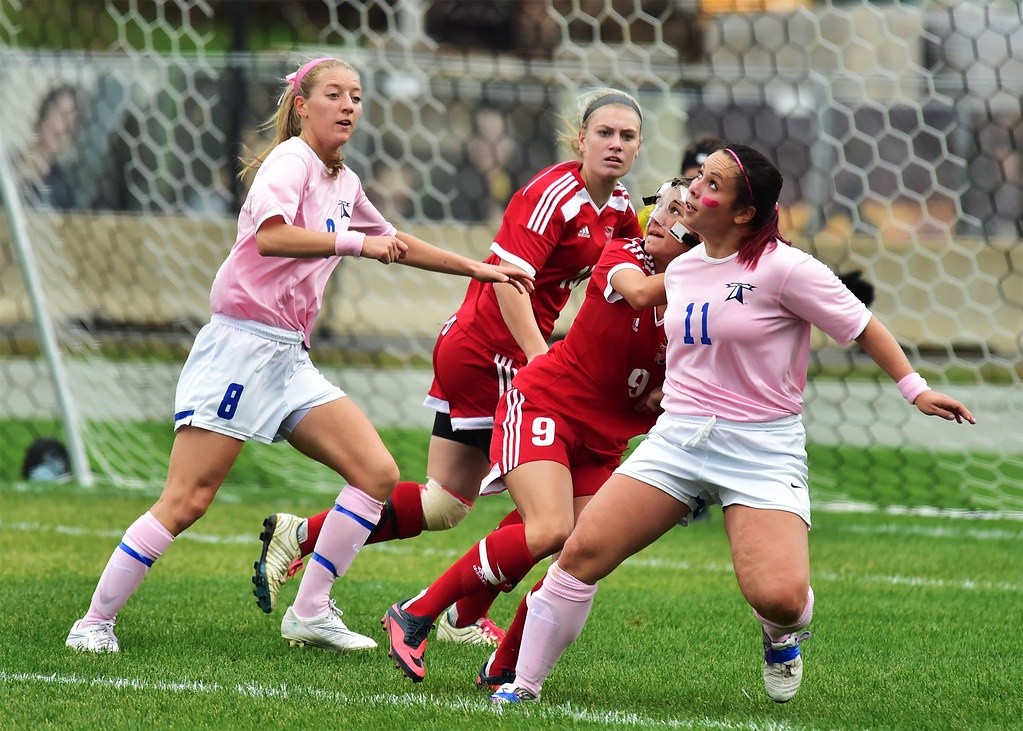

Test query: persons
[65,58,536,655]
[380,175,706,690]
[490,142,978,712]
[252,87,644,649]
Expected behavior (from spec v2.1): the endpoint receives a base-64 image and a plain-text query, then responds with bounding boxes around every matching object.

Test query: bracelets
[898,371,931,406]
[335,231,367,258]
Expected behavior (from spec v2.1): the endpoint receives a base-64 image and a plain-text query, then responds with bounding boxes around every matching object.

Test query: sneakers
[252,512,308,614]
[380,598,436,684]
[762,623,811,704]
[281,599,378,652]
[436,611,507,647]
[489,683,542,705]
[64,619,119,654]
[476,662,516,692]
